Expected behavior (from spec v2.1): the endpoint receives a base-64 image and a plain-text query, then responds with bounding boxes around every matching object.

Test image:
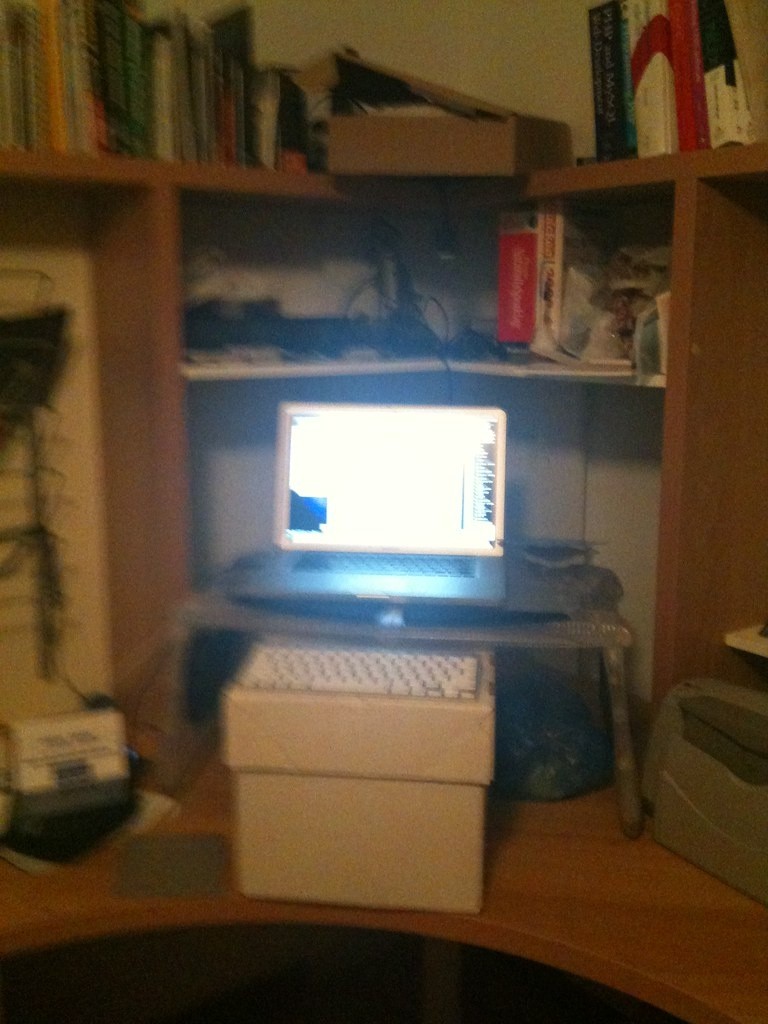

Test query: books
[1,0,768,353]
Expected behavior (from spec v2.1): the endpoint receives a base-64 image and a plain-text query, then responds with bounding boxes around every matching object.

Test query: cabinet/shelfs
[0,140,768,1024]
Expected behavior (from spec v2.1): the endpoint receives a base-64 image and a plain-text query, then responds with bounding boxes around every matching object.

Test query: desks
[163,594,649,847]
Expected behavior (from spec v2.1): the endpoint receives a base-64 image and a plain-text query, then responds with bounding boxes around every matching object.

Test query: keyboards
[241,641,479,702]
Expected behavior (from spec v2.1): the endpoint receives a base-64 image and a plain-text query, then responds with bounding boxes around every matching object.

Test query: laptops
[230,402,505,604]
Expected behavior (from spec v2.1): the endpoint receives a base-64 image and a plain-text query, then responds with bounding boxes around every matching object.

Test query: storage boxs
[0,681,132,793]
[301,39,575,178]
